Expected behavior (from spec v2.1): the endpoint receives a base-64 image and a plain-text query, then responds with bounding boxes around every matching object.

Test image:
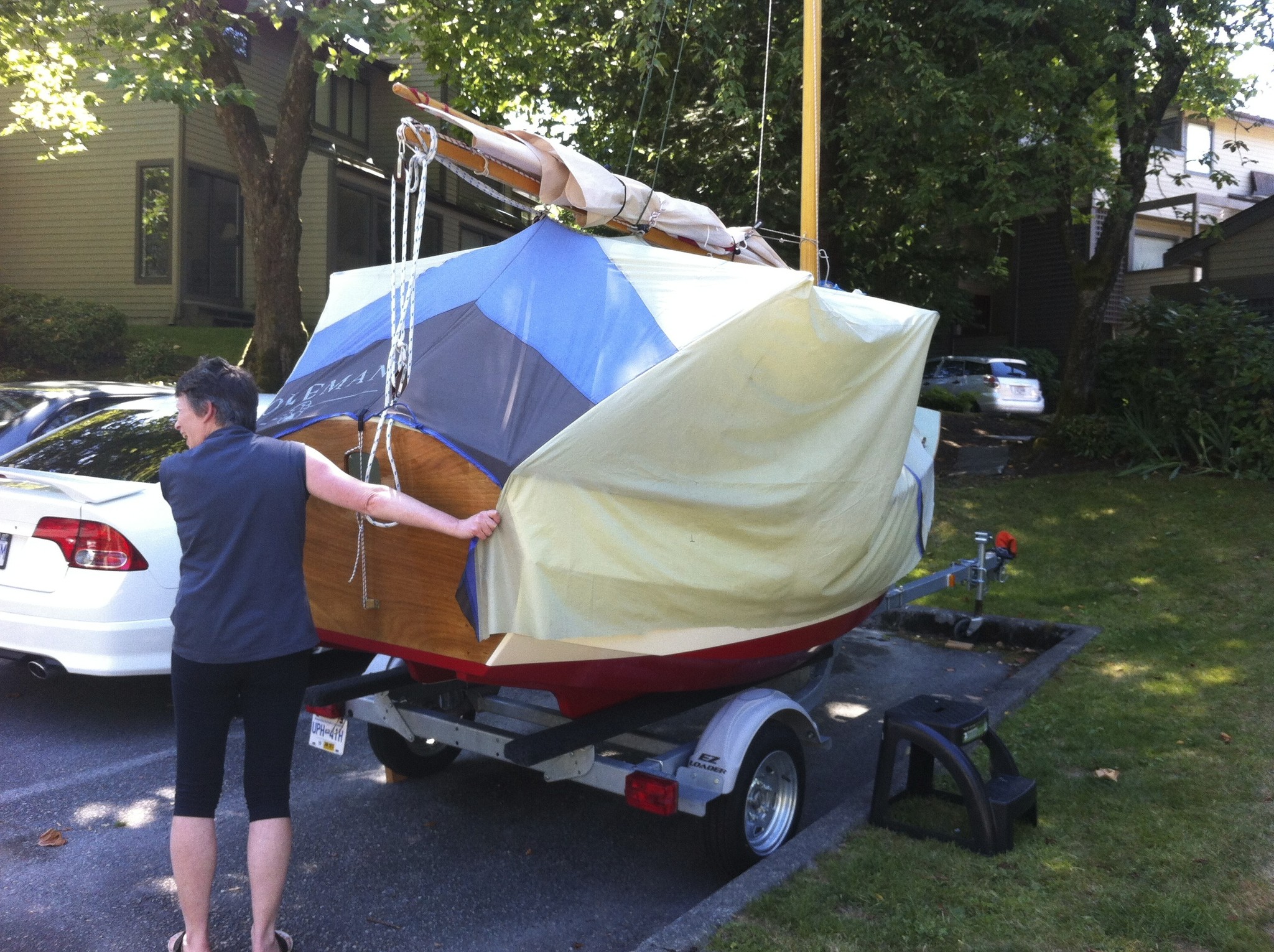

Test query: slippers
[273,929,293,952]
[168,931,190,952]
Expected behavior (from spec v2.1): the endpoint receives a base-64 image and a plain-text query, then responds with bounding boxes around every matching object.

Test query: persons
[159,357,501,952]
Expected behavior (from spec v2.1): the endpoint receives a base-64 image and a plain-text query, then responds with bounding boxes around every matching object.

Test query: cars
[0,392,277,678]
[919,355,1045,419]
[0,379,178,457]
